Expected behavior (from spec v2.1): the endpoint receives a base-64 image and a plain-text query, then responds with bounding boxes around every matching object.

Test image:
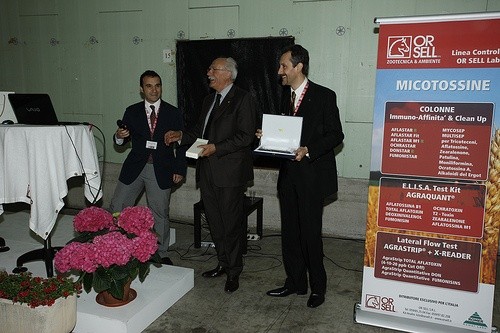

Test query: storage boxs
[185,137,208,159]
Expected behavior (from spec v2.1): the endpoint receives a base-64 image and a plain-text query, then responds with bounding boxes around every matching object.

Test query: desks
[0,124,103,279]
[194,196,263,249]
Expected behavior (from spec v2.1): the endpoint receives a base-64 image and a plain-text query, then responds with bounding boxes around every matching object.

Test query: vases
[102,281,130,304]
[0,288,76,333]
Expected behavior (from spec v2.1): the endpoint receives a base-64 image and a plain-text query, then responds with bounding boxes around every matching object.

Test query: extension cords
[201,242,215,247]
[247,234,260,240]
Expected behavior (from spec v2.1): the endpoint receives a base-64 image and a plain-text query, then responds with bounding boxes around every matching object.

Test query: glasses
[207,67,226,72]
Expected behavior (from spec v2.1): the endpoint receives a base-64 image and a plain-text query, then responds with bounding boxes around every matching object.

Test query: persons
[108,69,186,267]
[254,44,345,307]
[164,57,256,294]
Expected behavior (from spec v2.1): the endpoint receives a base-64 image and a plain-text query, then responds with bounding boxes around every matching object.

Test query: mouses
[2,120,14,125]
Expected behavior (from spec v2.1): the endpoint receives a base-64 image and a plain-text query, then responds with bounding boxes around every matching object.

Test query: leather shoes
[205,263,225,278]
[225,272,239,294]
[307,291,325,307]
[162,257,174,266]
[267,285,308,297]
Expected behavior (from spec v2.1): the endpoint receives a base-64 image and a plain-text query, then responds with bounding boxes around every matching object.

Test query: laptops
[8,93,80,125]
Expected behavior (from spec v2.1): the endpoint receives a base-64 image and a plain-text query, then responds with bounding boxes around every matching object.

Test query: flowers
[0,271,82,309]
[55,205,158,302]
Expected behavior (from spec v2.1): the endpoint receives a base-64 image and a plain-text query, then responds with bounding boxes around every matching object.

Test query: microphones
[117,119,131,141]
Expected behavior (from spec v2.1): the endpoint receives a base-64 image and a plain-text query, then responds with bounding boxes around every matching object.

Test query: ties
[214,94,222,111]
[291,91,296,116]
[150,105,156,129]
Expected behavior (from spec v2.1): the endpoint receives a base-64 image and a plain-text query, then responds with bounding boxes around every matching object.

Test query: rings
[122,133,124,136]
